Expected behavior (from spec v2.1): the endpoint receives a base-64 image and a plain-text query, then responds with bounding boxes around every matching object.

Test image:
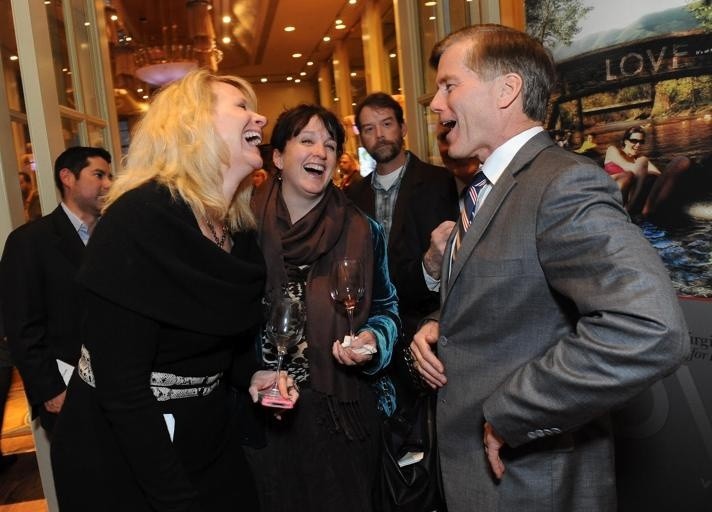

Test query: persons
[604,126,662,216]
[572,132,598,154]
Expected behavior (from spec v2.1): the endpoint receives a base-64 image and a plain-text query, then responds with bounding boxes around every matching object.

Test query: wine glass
[256,296,305,402]
[328,258,373,357]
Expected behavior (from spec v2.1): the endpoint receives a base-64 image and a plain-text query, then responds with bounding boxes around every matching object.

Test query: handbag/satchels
[371,389,441,511]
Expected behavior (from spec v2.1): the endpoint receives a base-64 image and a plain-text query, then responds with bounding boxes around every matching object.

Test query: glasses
[627,138,645,145]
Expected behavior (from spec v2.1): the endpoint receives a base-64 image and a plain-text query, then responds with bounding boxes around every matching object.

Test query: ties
[449,172,489,268]
